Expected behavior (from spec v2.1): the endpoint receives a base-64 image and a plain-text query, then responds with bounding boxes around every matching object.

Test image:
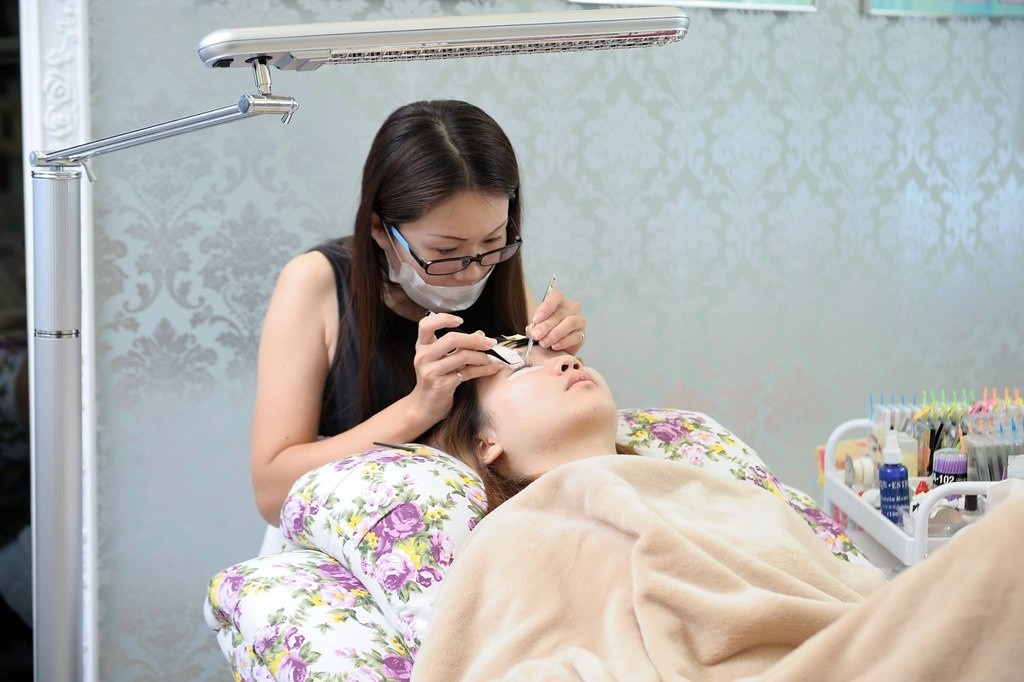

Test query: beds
[203,496,875,682]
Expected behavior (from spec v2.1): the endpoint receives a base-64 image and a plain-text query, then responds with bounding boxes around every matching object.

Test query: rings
[578,330,585,344]
[455,370,464,382]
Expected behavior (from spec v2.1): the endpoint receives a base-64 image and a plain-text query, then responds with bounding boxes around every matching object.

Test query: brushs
[373,442,436,457]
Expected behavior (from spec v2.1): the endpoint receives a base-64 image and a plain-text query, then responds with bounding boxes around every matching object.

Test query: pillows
[281,407,795,663]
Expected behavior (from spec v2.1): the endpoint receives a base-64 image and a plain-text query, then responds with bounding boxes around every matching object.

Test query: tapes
[843,455,876,488]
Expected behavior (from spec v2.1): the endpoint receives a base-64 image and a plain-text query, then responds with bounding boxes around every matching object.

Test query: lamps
[32,5,691,682]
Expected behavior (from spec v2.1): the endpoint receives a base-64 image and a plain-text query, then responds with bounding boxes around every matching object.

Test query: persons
[251,99,594,559]
[430,334,1024,682]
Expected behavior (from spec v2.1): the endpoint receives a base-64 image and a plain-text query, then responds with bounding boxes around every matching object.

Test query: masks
[381,221,496,313]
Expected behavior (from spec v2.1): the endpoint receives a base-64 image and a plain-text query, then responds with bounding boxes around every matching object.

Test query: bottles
[878,433,910,524]
[930,448,969,510]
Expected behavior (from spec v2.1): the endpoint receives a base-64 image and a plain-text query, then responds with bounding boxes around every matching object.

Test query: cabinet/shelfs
[823,415,1003,583]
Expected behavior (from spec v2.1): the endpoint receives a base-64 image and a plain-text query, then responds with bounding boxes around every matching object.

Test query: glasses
[390,213,523,276]
